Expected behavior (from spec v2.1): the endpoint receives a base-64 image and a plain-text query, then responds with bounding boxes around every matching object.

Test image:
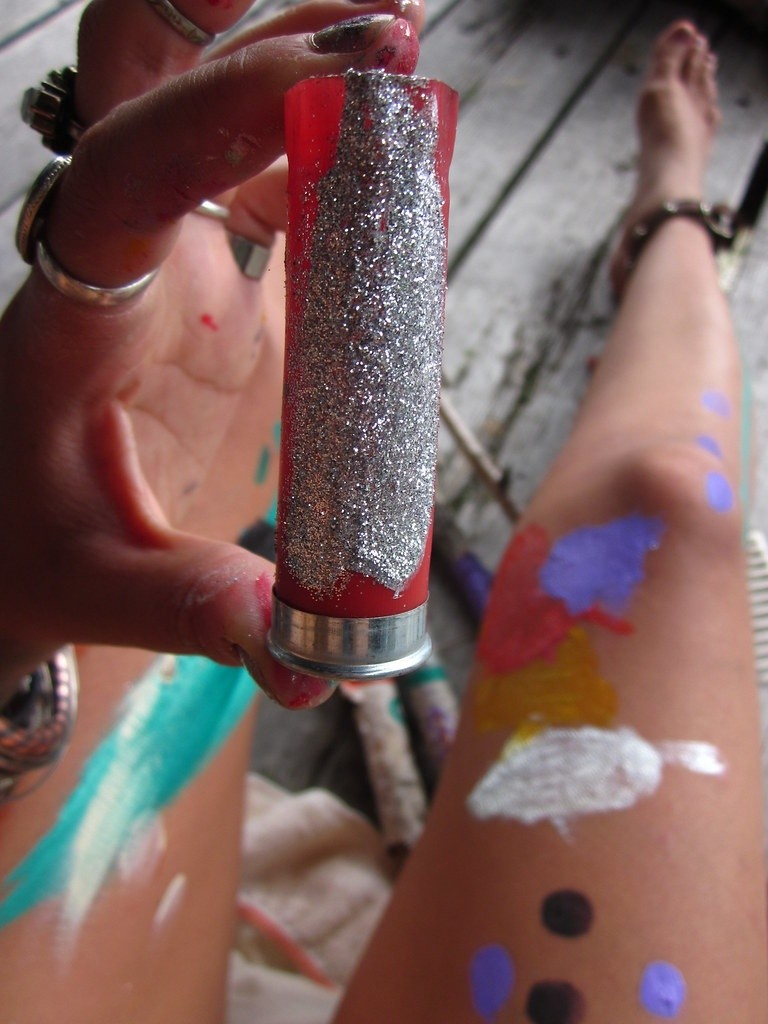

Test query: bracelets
[0,643,80,804]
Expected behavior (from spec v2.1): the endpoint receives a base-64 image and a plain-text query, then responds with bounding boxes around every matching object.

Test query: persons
[0,0,768,1024]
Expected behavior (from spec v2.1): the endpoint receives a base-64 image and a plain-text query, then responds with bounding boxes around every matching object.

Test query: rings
[227,227,273,279]
[192,198,230,218]
[149,0,214,51]
[16,152,165,307]
[20,66,91,154]
